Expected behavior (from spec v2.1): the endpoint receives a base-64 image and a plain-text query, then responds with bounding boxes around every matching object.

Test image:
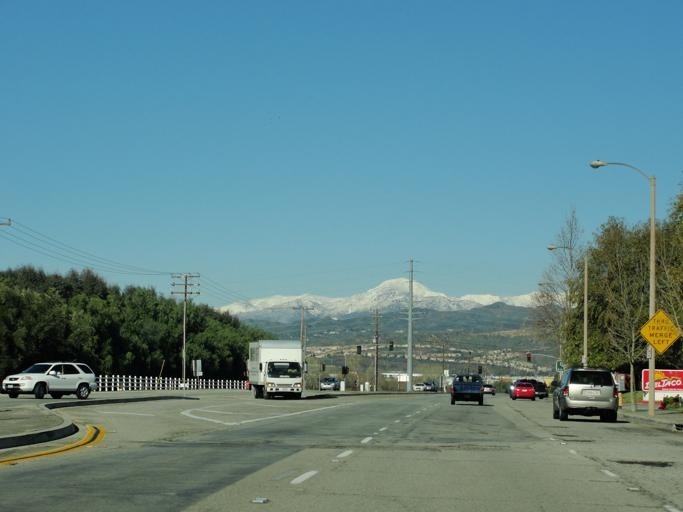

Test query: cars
[482,384,496,396]
[320,376,340,391]
[422,378,440,392]
[412,382,426,392]
[505,379,548,401]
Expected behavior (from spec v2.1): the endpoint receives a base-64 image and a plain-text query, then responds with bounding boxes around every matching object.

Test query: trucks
[243,339,307,399]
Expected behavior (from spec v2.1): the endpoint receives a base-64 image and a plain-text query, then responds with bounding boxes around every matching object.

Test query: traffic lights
[525,352,531,363]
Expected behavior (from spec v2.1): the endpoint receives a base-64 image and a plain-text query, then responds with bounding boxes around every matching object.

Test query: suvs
[549,367,619,424]
[2,359,98,400]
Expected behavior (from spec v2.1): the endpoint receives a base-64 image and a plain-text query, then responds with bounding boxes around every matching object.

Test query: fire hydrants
[617,391,625,409]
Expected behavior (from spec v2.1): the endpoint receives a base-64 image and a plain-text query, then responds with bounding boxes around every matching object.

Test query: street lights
[545,243,590,367]
[589,159,658,416]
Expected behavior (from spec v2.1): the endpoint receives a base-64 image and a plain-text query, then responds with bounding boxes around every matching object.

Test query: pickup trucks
[448,373,484,406]
